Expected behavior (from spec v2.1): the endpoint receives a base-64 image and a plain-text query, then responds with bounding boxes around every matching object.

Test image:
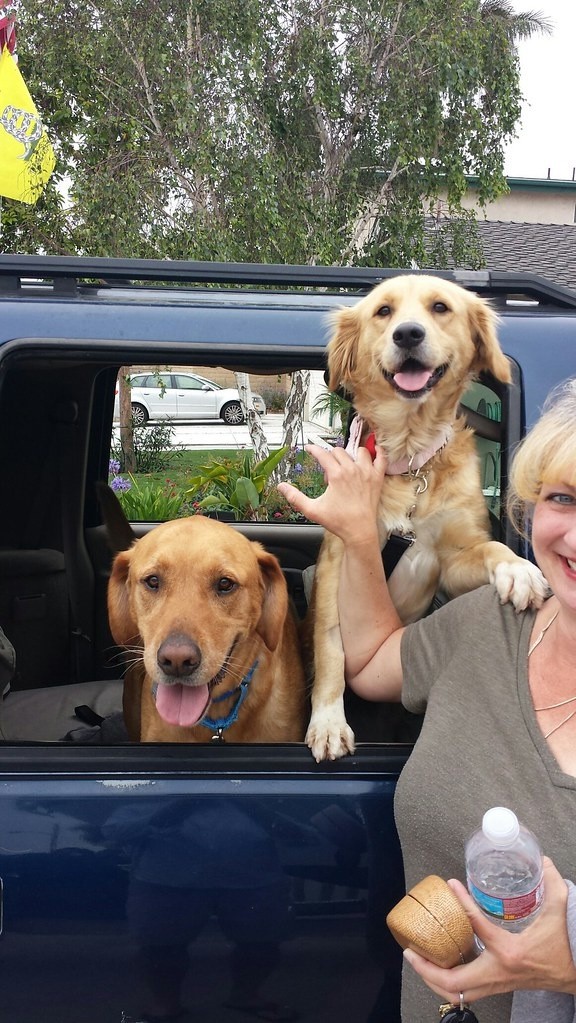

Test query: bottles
[466,807,544,952]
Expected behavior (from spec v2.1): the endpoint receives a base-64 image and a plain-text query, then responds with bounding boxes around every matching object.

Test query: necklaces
[527,612,576,740]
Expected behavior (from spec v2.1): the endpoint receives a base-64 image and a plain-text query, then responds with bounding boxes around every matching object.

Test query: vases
[204,511,235,520]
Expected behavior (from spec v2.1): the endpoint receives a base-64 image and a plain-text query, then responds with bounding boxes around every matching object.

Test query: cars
[115,372,267,426]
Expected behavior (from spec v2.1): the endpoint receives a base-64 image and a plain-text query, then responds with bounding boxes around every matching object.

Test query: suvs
[0,254,572,1022]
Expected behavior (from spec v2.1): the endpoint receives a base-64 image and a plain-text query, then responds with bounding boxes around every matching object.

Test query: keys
[439,1002,479,1023]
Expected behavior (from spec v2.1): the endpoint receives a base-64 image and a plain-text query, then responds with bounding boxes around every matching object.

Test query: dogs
[106,514,309,744]
[299,272,549,764]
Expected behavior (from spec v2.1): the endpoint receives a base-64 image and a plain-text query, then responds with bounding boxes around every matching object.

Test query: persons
[273,380,576,1023]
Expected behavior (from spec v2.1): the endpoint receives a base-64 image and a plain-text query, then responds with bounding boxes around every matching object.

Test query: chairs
[0,627,124,743]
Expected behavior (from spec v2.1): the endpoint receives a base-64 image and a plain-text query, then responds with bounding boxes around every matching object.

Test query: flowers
[190,488,233,514]
